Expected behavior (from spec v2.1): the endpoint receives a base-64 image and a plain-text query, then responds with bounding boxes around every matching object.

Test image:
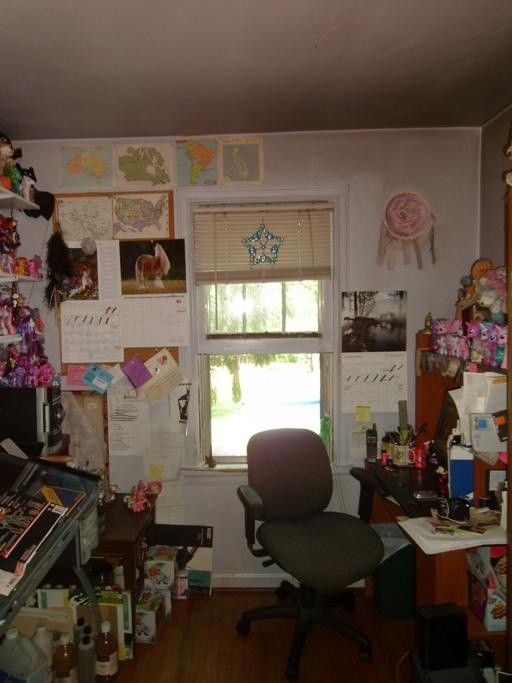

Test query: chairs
[236,428,384,681]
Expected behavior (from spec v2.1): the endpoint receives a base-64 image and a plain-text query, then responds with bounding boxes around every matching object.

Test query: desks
[91,494,155,644]
[365,328,512,672]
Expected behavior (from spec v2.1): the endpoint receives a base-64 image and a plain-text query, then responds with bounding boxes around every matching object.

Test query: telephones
[365,423,378,464]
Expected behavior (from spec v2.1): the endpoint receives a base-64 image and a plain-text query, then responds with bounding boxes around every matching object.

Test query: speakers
[414,602,469,669]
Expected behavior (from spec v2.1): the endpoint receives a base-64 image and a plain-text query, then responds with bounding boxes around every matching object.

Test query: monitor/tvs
[433,386,459,471]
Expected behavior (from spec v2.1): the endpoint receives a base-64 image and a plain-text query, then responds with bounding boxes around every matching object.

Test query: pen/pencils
[385,426,413,446]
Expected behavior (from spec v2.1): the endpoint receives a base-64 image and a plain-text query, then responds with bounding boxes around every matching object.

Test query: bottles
[53,633,78,683]
[0,628,48,682]
[73,617,94,682]
[30,624,55,683]
[415,428,427,470]
[94,619,119,683]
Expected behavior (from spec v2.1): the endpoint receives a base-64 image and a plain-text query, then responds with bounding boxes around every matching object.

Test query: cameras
[440,497,471,522]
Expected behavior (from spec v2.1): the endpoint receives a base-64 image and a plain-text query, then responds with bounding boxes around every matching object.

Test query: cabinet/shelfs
[0,187,43,344]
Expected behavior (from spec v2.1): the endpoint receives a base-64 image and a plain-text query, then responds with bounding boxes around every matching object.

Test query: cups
[392,445,415,467]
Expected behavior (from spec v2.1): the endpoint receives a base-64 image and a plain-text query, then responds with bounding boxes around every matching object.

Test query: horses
[135,243,172,289]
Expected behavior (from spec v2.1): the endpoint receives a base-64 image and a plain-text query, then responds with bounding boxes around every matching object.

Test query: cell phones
[413,489,437,501]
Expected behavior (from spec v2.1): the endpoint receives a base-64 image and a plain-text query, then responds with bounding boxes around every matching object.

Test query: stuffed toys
[431,259,508,371]
[0,135,56,389]
